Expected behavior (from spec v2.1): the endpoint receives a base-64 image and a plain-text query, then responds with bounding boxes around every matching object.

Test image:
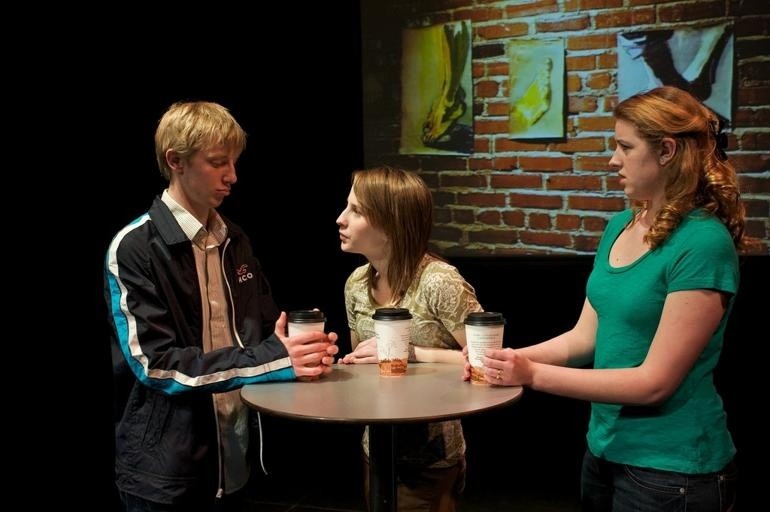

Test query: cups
[369,306,413,377]
[287,307,326,382]
[464,308,506,386]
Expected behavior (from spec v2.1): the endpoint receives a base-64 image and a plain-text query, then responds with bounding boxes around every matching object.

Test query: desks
[239,348,522,512]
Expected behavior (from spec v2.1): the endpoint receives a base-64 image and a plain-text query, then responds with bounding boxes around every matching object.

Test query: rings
[496,369,501,380]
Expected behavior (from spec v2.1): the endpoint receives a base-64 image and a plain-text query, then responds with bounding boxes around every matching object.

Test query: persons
[101,102,342,510]
[322,166,489,511]
[459,84,765,510]
